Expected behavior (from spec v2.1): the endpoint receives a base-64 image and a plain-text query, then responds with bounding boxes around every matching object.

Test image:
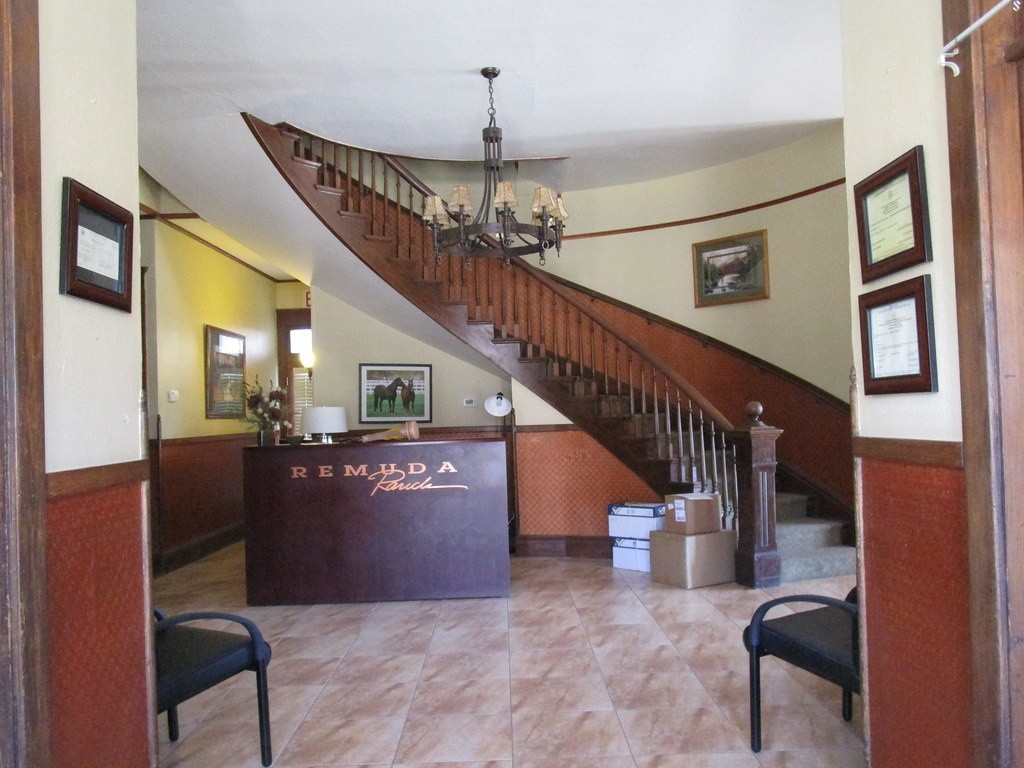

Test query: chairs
[742,585,860,752]
[152,608,275,767]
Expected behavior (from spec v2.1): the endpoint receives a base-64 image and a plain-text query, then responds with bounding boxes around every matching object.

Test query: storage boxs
[664,492,722,535]
[607,516,666,541]
[612,546,650,573]
[649,529,737,590]
[610,536,649,549]
[608,501,665,518]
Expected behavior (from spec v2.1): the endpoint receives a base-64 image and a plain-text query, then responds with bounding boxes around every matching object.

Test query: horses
[401,379,415,414]
[374,377,406,413]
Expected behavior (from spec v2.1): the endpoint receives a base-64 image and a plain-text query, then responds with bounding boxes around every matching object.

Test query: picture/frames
[691,228,771,309]
[59,174,135,316]
[853,143,934,284]
[358,362,433,425]
[858,274,940,396]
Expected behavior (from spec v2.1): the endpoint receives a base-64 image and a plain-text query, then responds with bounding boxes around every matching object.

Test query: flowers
[242,374,293,430]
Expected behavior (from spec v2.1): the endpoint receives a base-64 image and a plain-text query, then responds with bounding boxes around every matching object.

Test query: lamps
[484,391,514,438]
[299,349,316,382]
[299,404,349,443]
[205,324,247,420]
[422,66,570,271]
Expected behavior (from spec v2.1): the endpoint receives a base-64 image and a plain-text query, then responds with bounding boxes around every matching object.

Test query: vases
[257,430,280,446]
